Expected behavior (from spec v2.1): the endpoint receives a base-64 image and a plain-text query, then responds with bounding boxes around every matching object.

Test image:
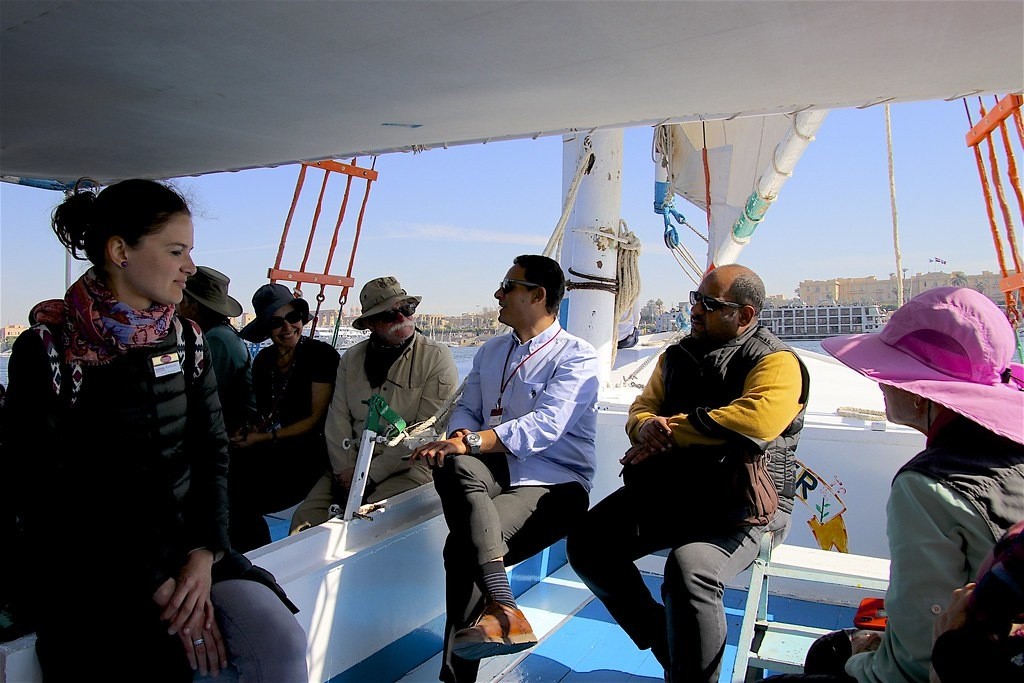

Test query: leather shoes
[452,601,537,660]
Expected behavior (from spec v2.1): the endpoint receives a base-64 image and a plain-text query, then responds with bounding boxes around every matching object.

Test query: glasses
[500,279,541,294]
[381,302,416,324]
[689,290,743,313]
[264,309,303,331]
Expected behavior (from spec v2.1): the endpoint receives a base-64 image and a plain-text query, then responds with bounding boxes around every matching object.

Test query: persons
[227,284,342,554]
[566,264,811,682]
[175,266,258,430]
[762,286,1024,683]
[407,254,599,682]
[0,179,308,683]
[929,521,1024,683]
[289,276,458,536]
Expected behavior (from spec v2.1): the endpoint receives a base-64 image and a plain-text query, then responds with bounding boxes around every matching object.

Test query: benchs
[628,545,891,630]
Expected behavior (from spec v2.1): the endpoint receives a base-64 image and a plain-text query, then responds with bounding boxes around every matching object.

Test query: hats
[183,265,244,318]
[821,286,1023,445]
[236,284,314,344]
[352,276,423,330]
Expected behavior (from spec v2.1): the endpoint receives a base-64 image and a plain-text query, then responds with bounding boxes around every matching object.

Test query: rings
[194,639,203,645]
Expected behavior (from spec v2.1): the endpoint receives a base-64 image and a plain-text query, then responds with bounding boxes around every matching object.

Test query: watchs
[465,432,482,456]
[272,432,279,443]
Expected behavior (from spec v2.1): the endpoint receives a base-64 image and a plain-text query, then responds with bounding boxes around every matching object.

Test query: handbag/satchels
[621,444,751,542]
